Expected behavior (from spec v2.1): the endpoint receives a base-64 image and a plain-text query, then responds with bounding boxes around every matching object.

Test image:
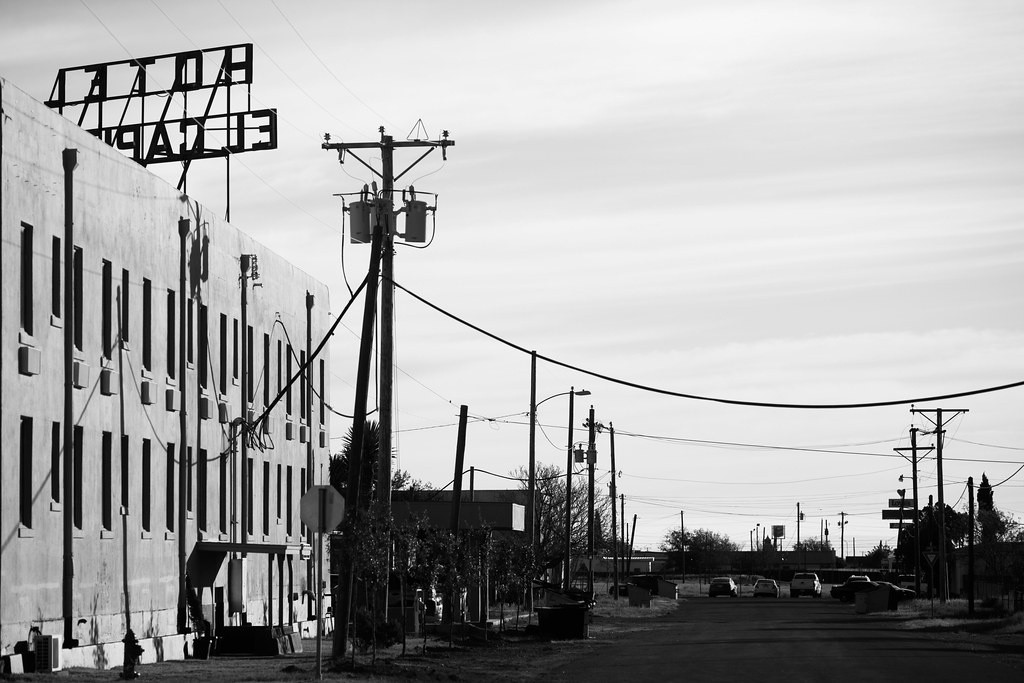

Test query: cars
[708,577,738,598]
[752,578,780,598]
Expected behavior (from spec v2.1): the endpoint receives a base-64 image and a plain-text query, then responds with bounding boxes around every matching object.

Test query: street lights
[525,350,592,615]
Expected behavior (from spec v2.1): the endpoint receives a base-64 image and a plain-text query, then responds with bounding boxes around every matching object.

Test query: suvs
[789,572,824,599]
[830,574,917,611]
